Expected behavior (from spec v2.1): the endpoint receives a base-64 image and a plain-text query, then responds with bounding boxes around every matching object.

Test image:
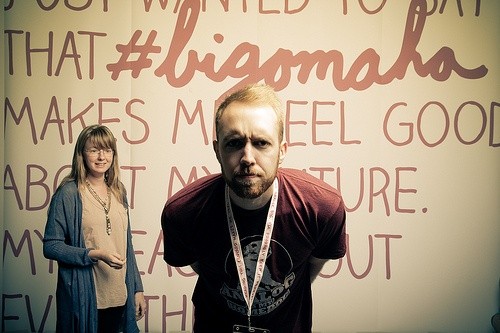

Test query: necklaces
[84,179,114,236]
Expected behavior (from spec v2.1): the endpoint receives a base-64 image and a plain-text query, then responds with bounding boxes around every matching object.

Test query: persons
[159,83,347,333]
[42,123,148,333]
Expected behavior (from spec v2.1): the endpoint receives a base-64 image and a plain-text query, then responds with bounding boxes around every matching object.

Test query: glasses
[85,148,114,156]
